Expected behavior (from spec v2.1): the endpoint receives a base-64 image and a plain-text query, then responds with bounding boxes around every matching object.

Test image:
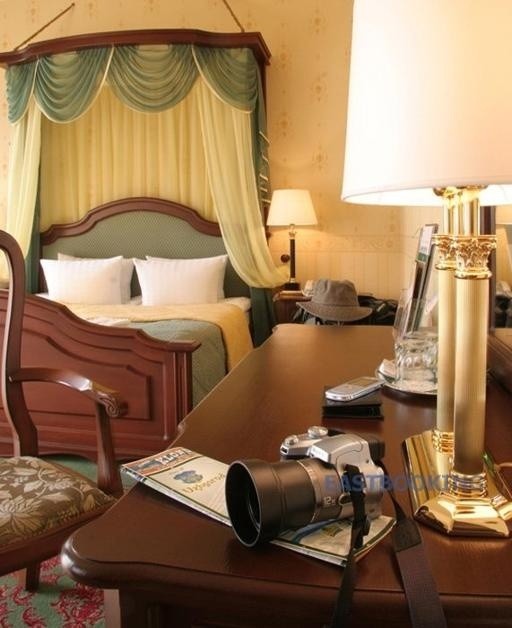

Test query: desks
[60,323,512,628]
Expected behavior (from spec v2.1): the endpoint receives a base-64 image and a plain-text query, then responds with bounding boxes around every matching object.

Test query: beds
[1,29,290,465]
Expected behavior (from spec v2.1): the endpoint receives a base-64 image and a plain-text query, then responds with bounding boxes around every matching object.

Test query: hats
[296,278,374,326]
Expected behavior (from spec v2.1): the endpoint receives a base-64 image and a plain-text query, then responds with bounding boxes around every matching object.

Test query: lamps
[267,189,318,295]
[340,1,511,539]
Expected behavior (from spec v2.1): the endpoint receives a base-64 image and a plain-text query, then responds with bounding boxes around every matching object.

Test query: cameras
[225,425,384,549]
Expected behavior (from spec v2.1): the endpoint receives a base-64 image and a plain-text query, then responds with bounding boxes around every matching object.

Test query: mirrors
[479,205,511,390]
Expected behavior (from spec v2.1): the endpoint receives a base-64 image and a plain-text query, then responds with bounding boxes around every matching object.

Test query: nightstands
[273,284,310,321]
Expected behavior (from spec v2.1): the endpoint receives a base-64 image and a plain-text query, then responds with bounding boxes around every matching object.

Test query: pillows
[39,252,229,306]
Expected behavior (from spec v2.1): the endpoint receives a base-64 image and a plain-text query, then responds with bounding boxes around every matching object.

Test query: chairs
[0,229,123,592]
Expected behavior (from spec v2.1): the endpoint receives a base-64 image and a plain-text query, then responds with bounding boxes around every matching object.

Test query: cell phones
[325,376,385,401]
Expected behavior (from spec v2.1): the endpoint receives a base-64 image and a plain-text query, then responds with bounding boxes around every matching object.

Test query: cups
[395,330,439,391]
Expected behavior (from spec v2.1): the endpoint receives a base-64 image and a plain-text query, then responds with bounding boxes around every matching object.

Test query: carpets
[1,456,122,628]
